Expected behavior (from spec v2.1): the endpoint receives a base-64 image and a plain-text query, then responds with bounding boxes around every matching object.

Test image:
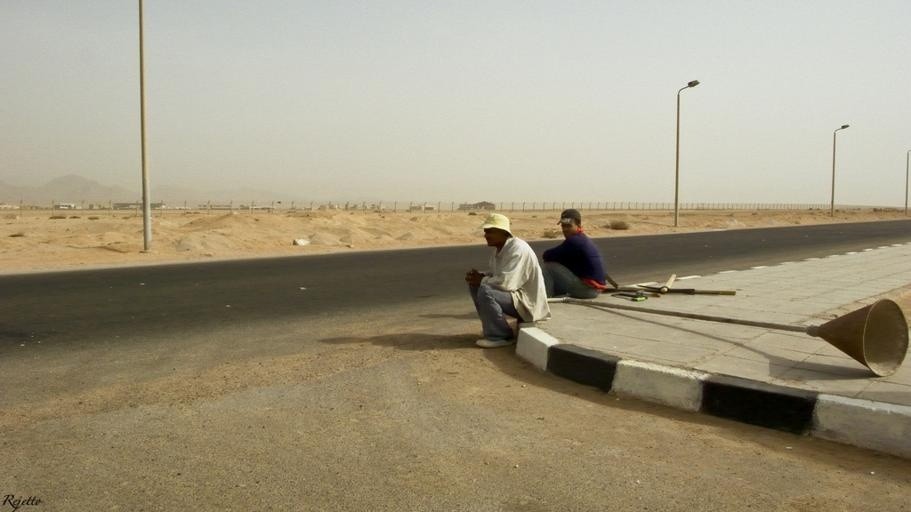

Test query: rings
[469,275,473,279]
[470,271,473,274]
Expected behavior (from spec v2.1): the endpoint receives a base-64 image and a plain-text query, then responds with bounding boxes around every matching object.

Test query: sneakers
[476,332,517,348]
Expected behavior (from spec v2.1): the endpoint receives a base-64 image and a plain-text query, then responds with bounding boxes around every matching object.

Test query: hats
[481,212,515,240]
[556,208,582,226]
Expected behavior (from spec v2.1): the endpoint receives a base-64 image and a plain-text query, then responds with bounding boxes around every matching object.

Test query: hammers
[637,273,695,294]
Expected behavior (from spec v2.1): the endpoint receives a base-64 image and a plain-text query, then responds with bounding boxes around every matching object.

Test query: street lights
[831,124,850,217]
[674,79,700,226]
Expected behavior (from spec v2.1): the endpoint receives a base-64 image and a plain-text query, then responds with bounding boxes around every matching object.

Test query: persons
[465,212,553,349]
[541,208,609,300]
